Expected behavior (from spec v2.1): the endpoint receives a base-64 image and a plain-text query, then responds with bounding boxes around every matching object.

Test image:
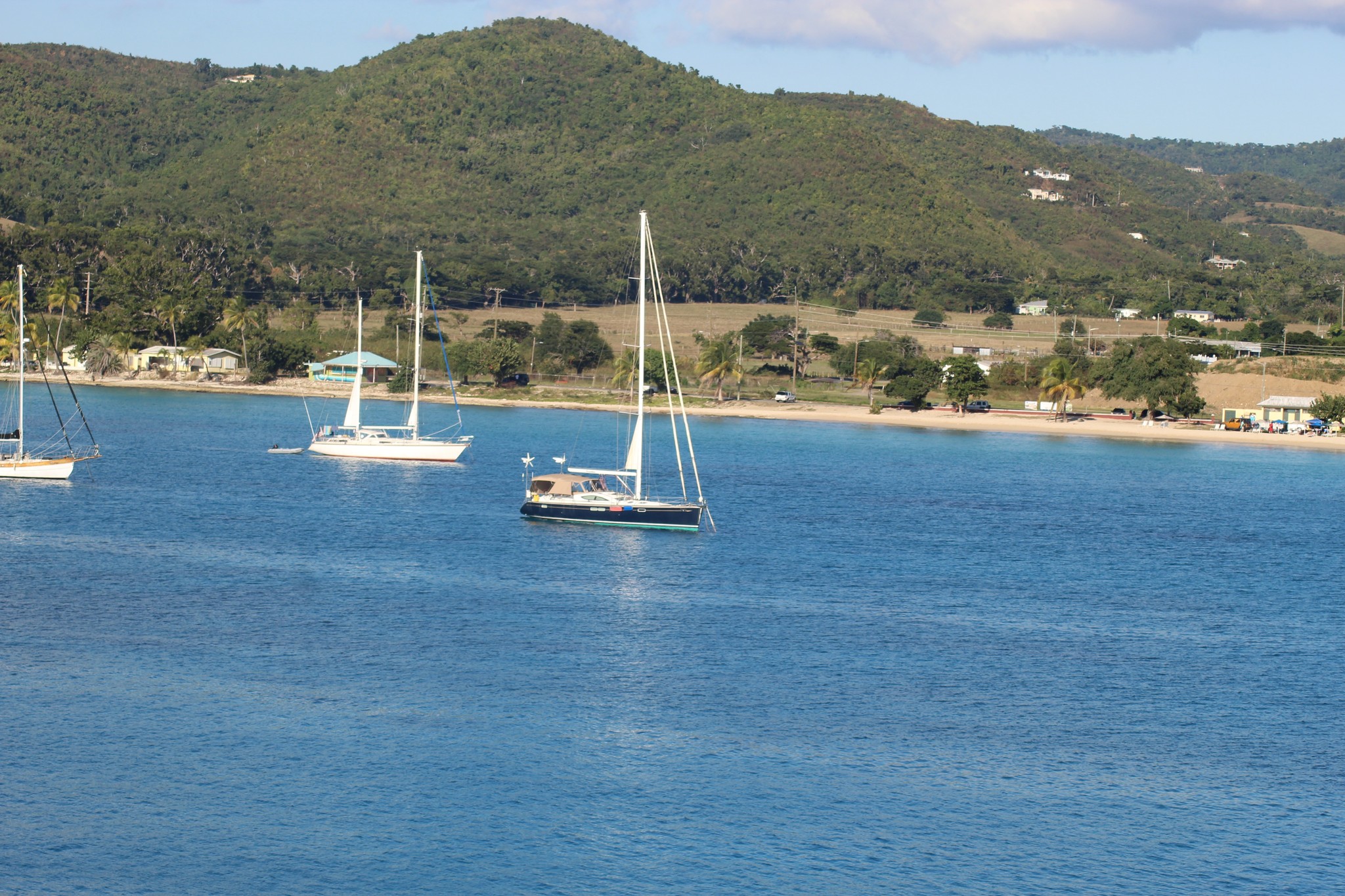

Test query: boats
[267,444,305,454]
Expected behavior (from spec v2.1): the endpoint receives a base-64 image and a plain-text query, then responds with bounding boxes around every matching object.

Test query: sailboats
[0,264,104,480]
[519,206,718,533]
[302,249,475,463]
